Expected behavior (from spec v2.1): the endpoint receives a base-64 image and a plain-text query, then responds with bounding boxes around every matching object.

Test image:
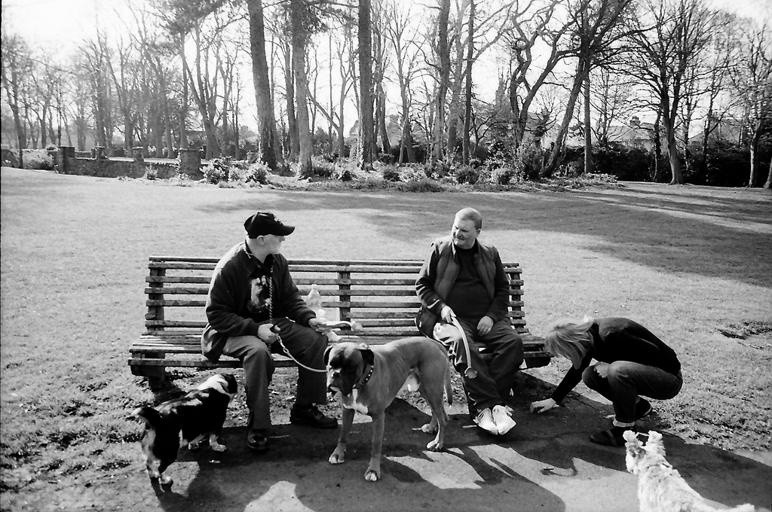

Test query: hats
[244,212,295,236]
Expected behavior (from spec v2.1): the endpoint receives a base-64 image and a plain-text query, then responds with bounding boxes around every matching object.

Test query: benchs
[126,251,556,413]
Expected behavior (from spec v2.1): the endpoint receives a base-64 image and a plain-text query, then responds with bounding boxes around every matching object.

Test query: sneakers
[473,405,516,436]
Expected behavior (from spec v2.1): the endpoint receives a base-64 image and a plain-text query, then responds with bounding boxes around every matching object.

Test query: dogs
[131,373,238,500]
[323,335,452,482]
[622,430,756,512]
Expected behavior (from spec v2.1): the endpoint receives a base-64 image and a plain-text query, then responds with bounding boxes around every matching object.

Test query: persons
[530,316,682,443]
[200,212,337,448]
[414,208,523,435]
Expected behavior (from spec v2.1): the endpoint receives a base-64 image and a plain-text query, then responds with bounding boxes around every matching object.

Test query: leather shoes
[589,429,626,446]
[246,431,269,450]
[290,406,338,429]
